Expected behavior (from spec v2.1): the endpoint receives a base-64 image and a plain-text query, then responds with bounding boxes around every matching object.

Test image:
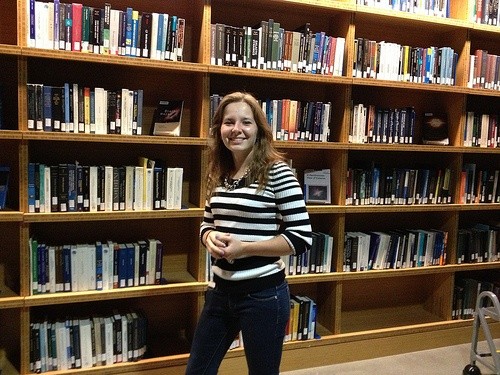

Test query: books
[455,277,500,321]
[29,306,151,373]
[211,95,500,151]
[209,1,498,92]
[279,226,500,275]
[230,291,318,349]
[26,81,145,134]
[289,156,499,205]
[22,4,188,62]
[151,97,184,136]
[27,236,166,292]
[0,157,189,210]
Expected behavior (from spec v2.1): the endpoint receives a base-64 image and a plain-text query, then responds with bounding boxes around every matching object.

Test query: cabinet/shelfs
[0,0,500,374]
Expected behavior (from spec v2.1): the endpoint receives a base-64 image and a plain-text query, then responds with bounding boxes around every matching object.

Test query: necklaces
[223,162,256,191]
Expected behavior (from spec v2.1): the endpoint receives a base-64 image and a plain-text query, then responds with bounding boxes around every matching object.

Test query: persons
[183,88,315,374]
